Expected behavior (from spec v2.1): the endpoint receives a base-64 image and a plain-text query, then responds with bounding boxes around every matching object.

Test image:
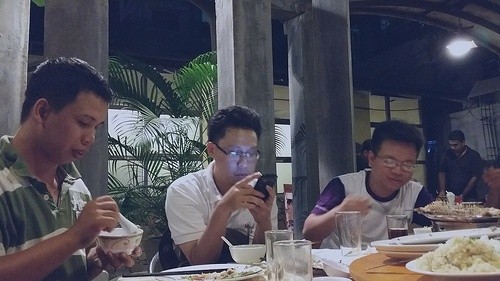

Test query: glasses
[210,140,261,161]
[449,140,463,146]
[374,154,416,172]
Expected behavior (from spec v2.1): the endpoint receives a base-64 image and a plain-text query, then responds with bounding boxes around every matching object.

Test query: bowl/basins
[96,228,145,257]
[229,243,267,264]
[318,257,351,277]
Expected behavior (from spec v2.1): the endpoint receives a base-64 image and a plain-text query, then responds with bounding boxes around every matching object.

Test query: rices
[416,234,500,273]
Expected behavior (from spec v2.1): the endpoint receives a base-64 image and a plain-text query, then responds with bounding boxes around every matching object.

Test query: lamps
[447,24,478,58]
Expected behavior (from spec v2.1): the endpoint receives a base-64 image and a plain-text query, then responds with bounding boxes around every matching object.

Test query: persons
[437,129,483,203]
[356,137,371,172]
[164,105,280,266]
[0,55,142,281]
[302,117,435,249]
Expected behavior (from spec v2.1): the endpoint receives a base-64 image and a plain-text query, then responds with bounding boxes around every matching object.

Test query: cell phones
[249,174,276,206]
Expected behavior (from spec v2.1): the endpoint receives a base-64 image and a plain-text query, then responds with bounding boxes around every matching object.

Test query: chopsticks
[121,268,234,278]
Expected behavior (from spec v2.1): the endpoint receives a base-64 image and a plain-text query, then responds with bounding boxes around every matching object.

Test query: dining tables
[247,248,500,281]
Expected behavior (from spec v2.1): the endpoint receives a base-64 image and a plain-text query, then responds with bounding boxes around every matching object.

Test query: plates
[155,263,263,281]
[308,244,370,281]
[370,202,500,281]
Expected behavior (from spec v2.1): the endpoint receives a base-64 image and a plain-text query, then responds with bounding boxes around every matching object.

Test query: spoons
[116,212,139,235]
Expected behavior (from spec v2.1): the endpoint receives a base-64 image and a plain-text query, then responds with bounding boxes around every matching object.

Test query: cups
[333,210,362,260]
[271,238,314,281]
[385,213,408,240]
[265,230,295,281]
